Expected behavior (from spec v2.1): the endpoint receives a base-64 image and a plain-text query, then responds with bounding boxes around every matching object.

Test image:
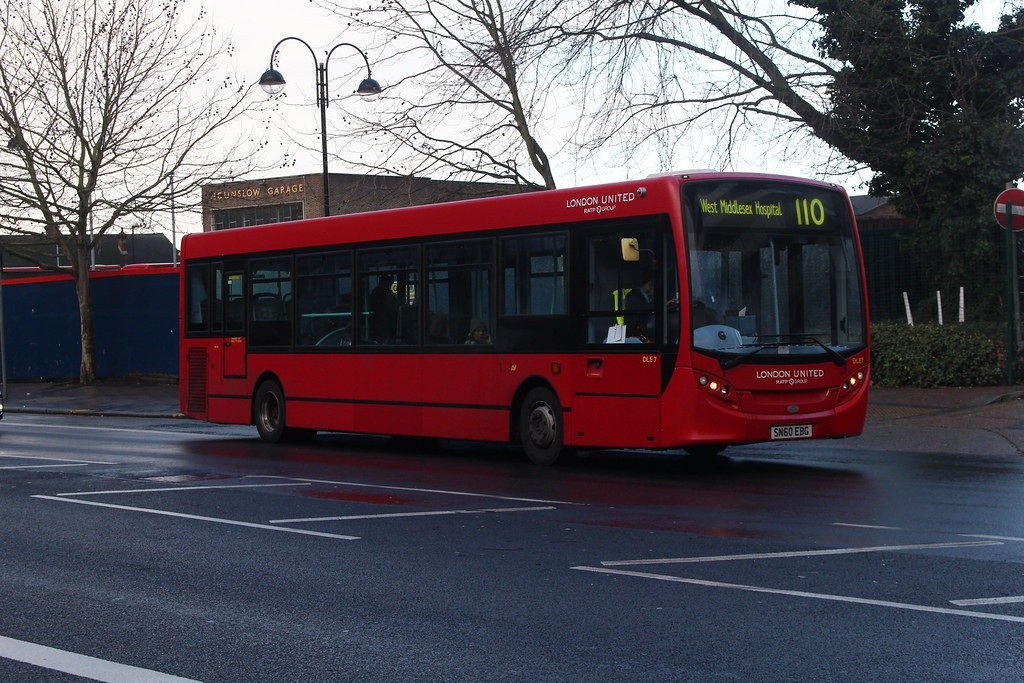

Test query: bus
[179,170,870,465]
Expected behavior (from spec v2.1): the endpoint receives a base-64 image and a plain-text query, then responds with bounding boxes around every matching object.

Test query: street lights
[165,172,177,268]
[131,225,139,263]
[258,37,383,217]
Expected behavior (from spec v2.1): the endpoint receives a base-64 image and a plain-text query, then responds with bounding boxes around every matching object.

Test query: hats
[383,271,394,283]
[468,318,489,338]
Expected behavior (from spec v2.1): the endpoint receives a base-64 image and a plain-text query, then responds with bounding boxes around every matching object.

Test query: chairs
[284,292,291,318]
[252,291,283,321]
[199,297,222,323]
[227,294,244,322]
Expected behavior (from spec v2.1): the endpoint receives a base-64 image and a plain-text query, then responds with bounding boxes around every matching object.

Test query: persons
[371,275,393,296]
[624,269,655,338]
[465,318,493,345]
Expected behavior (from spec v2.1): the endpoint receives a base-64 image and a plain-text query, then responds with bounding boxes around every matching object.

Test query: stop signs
[994,189,1024,232]
